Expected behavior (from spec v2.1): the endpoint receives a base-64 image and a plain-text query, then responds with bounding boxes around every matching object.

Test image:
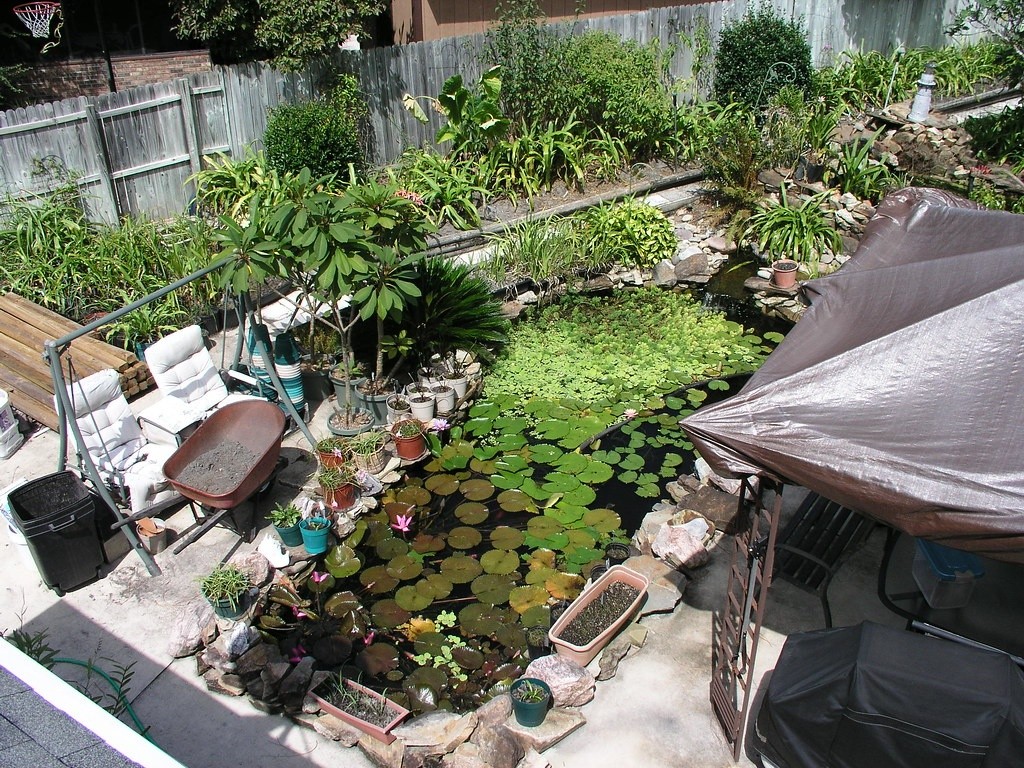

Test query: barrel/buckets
[135,518,167,554]
[0,388,23,457]
[248,324,305,415]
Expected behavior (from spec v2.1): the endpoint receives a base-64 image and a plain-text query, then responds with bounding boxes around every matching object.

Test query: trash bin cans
[6,469,105,597]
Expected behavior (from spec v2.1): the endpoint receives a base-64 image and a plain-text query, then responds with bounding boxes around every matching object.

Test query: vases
[770,259,798,285]
[137,518,167,554]
[311,503,333,524]
[352,432,385,473]
[800,157,823,182]
[590,564,607,581]
[328,406,375,436]
[318,478,355,508]
[391,419,427,461]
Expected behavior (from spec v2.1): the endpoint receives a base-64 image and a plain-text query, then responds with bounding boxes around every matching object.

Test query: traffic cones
[249,323,278,402]
[274,333,305,412]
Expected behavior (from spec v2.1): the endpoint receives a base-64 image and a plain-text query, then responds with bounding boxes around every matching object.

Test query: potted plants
[298,516,331,553]
[199,561,251,619]
[604,541,629,563]
[305,670,409,746]
[550,565,650,668]
[307,436,353,468]
[525,625,553,660]
[265,500,303,547]
[550,602,568,627]
[510,677,550,727]
[207,167,629,422]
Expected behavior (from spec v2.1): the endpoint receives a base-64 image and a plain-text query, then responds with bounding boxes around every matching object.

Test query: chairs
[749,490,875,628]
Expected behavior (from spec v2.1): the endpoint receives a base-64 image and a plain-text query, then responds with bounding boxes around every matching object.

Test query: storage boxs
[912,538,985,609]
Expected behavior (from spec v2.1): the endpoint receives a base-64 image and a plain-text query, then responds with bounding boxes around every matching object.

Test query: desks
[877,531,1024,667]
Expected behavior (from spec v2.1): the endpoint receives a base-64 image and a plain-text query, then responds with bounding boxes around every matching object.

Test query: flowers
[395,418,431,445]
[333,427,392,462]
[317,502,326,512]
[313,464,366,510]
[796,93,849,156]
[214,197,416,424]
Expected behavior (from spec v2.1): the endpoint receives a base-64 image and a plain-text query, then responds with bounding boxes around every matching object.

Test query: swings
[65,261,268,515]
